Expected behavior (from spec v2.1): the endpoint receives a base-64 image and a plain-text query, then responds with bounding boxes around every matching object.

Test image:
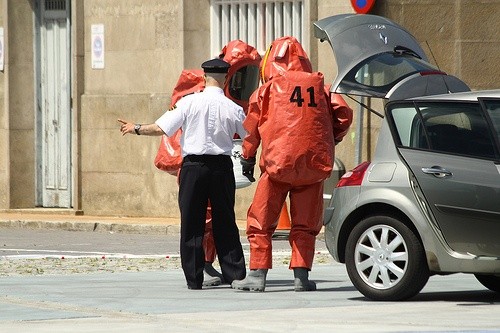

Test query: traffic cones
[270,201,292,240]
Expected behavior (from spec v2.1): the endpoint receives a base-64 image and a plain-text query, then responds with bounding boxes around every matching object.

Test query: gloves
[240,157,255,182]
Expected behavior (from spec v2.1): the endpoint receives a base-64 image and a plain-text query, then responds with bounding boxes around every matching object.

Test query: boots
[231,269,267,292]
[294,268,316,291]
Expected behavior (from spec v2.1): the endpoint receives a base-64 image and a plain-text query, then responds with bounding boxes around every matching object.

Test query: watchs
[134,124,141,135]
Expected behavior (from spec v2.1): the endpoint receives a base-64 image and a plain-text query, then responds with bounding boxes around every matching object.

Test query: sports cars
[313,12,500,300]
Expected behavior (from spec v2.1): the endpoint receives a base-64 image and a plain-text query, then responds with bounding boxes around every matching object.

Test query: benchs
[428,123,496,159]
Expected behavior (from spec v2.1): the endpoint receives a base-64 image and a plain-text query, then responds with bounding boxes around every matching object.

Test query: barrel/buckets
[230,139,252,189]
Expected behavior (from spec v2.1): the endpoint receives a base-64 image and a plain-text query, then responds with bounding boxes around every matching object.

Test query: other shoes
[205,263,225,283]
[188,284,202,289]
[203,270,221,286]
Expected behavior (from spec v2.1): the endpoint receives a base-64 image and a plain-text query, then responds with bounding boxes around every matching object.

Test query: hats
[201,59,230,73]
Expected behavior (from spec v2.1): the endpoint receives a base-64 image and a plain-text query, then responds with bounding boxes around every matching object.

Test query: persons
[154,40,262,286]
[231,36,353,291]
[118,59,247,290]
